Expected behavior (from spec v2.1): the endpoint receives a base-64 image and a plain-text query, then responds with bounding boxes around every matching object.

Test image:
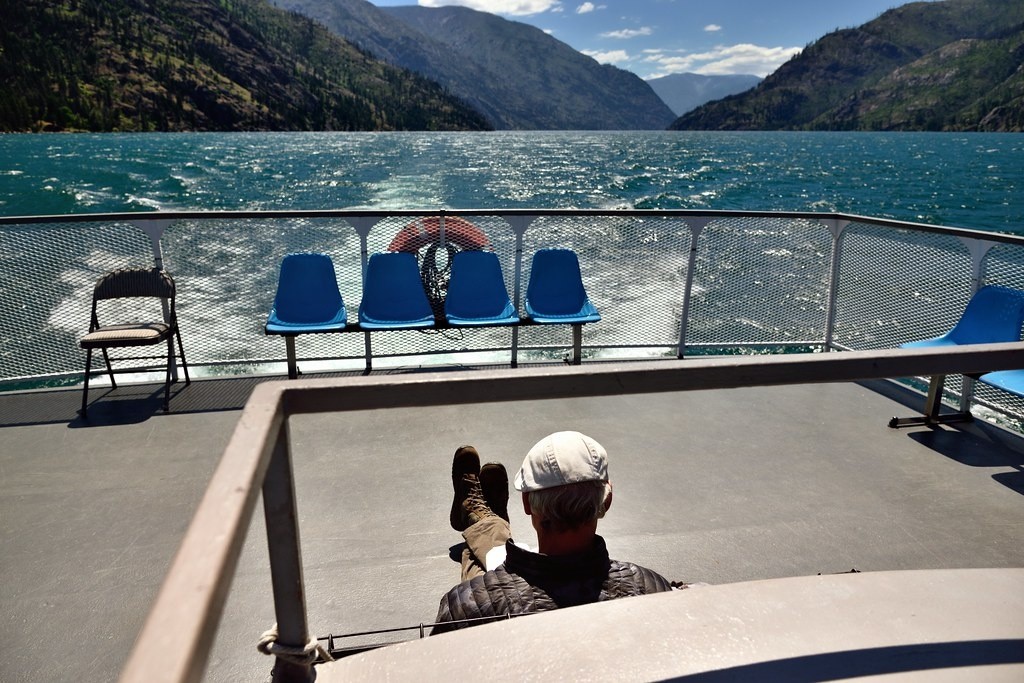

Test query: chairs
[262,248,346,380]
[888,285,1024,429]
[980,369,1024,399]
[358,252,435,331]
[524,248,603,364]
[79,268,190,415]
[447,251,520,329]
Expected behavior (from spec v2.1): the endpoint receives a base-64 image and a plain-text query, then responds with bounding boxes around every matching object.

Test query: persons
[427,430,673,636]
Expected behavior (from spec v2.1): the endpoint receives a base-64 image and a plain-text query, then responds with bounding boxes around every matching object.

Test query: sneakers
[449,446,498,532]
[479,463,510,526]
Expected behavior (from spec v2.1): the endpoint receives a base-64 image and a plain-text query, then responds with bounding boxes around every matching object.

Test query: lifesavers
[386,217,496,330]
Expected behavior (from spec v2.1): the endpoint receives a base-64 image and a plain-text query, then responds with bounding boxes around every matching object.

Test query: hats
[513,431,608,492]
